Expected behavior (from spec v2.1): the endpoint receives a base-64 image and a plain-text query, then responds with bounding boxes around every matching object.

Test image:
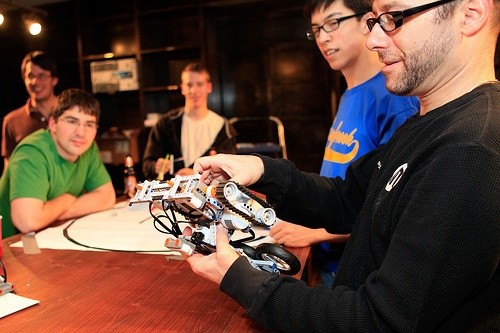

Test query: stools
[229,115,289,160]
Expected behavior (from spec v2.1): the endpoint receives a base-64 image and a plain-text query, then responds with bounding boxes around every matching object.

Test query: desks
[0,187,313,333]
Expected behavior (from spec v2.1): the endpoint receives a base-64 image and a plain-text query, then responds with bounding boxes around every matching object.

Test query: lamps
[21,13,43,36]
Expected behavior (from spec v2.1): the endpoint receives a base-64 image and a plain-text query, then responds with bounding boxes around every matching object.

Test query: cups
[0,215,3,274]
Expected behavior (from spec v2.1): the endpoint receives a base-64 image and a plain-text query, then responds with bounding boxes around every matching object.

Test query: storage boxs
[89,58,120,93]
[119,56,140,93]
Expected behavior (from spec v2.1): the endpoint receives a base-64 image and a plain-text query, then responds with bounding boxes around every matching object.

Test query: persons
[180,0,500,333]
[144,62,239,181]
[0,88,116,240]
[3,51,61,173]
[269,0,421,288]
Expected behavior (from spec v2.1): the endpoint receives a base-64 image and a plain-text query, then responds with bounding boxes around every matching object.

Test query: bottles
[123,153,138,199]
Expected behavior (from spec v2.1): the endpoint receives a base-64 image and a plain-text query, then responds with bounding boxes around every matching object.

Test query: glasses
[27,73,51,82]
[366,0,455,32]
[305,11,364,41]
[59,119,99,132]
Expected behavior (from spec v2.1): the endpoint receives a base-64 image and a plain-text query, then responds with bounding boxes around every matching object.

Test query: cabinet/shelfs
[71,0,335,174]
